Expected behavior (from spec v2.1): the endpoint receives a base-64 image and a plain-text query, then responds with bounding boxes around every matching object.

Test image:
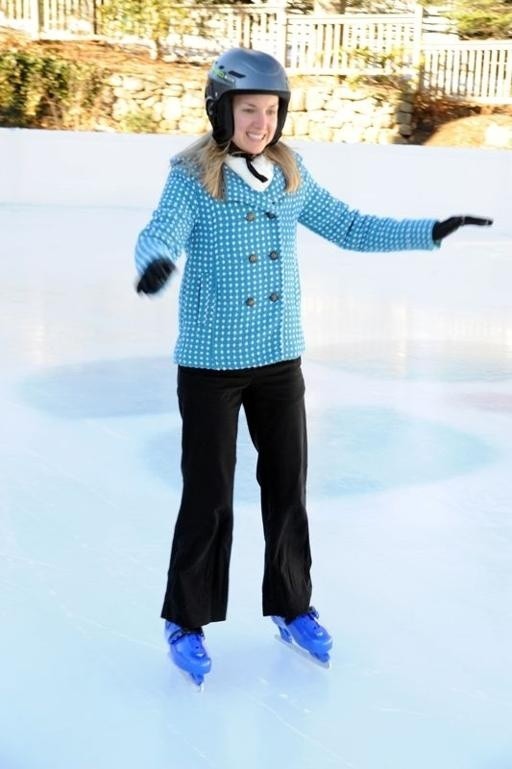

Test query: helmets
[204,46,293,146]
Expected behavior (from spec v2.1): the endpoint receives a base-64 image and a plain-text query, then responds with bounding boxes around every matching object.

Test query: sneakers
[164,619,212,675]
[273,608,334,654]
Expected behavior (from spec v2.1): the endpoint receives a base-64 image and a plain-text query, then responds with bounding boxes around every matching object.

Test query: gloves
[134,259,175,295]
[432,213,493,242]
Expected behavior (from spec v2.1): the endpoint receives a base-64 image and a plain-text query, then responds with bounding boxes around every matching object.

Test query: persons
[135,48,493,674]
